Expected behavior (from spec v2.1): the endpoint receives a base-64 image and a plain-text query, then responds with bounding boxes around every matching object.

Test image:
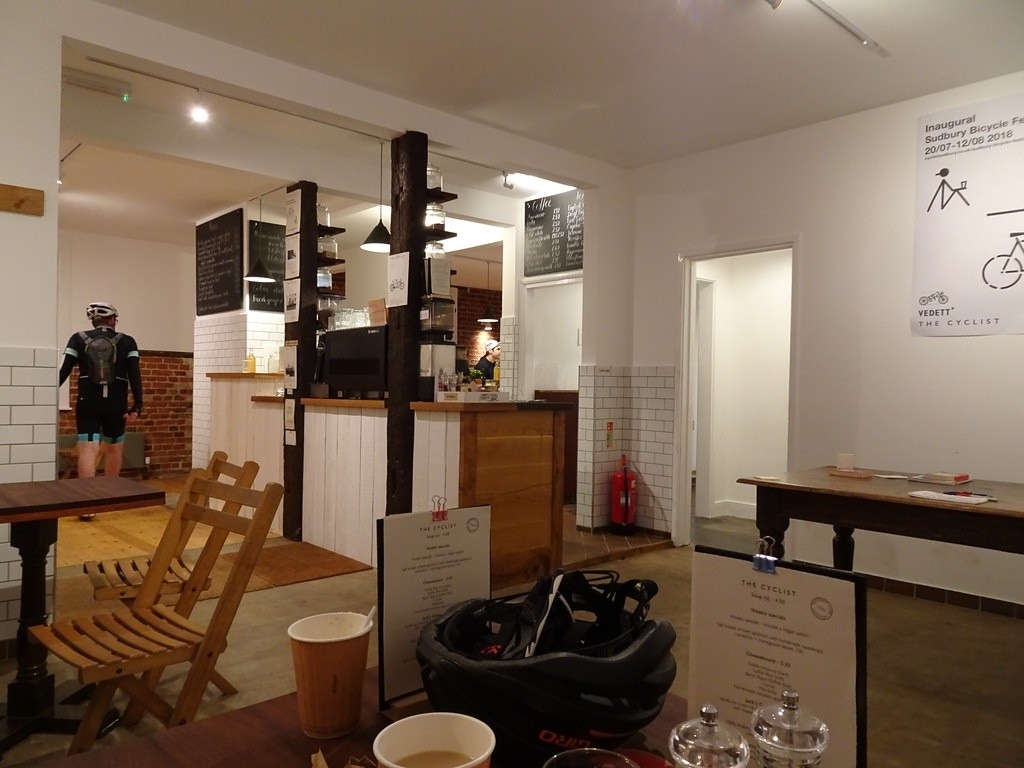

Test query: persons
[474,340,500,385]
[60,303,143,519]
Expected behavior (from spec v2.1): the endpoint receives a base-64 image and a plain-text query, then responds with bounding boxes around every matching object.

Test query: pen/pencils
[944,491,998,502]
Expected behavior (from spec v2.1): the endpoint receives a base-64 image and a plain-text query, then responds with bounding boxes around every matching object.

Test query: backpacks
[77,331,126,384]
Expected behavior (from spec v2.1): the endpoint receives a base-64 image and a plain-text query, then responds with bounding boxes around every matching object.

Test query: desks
[737,466,1024,570]
[0,477,167,757]
[28,667,691,768]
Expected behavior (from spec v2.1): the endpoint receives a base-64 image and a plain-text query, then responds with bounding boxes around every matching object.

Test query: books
[909,473,974,486]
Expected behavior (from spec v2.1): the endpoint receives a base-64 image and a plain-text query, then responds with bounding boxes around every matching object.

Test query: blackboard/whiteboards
[196,209,244,316]
[248,220,284,313]
[524,188,584,282]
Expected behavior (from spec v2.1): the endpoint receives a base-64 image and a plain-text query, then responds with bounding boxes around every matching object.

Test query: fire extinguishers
[609,455,638,536]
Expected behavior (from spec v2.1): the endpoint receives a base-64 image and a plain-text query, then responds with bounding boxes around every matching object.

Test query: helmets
[86,303,119,321]
[415,571,675,752]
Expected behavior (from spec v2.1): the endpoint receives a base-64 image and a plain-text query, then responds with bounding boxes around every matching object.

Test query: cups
[242,360,251,373]
[542,748,641,768]
[836,453,854,469]
[276,379,285,397]
[288,612,374,739]
[372,712,496,768]
[327,306,370,331]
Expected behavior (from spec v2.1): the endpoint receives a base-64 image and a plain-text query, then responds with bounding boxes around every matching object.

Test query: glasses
[113,318,118,324]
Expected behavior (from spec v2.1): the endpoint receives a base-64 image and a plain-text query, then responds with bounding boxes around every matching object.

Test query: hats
[485,340,500,353]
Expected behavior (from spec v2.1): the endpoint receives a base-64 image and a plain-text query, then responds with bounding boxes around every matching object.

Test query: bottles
[247,347,256,373]
[317,267,332,288]
[669,704,750,768]
[317,203,330,226]
[425,202,446,230]
[427,162,443,191]
[425,240,446,259]
[317,234,337,259]
[750,691,829,768]
[438,360,500,393]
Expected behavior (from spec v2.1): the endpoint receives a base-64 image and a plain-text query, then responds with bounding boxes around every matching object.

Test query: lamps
[244,198,276,283]
[360,143,391,253]
[477,262,498,322]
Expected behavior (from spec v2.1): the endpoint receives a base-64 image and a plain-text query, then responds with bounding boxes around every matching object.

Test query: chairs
[28,468,283,752]
[82,450,261,613]
